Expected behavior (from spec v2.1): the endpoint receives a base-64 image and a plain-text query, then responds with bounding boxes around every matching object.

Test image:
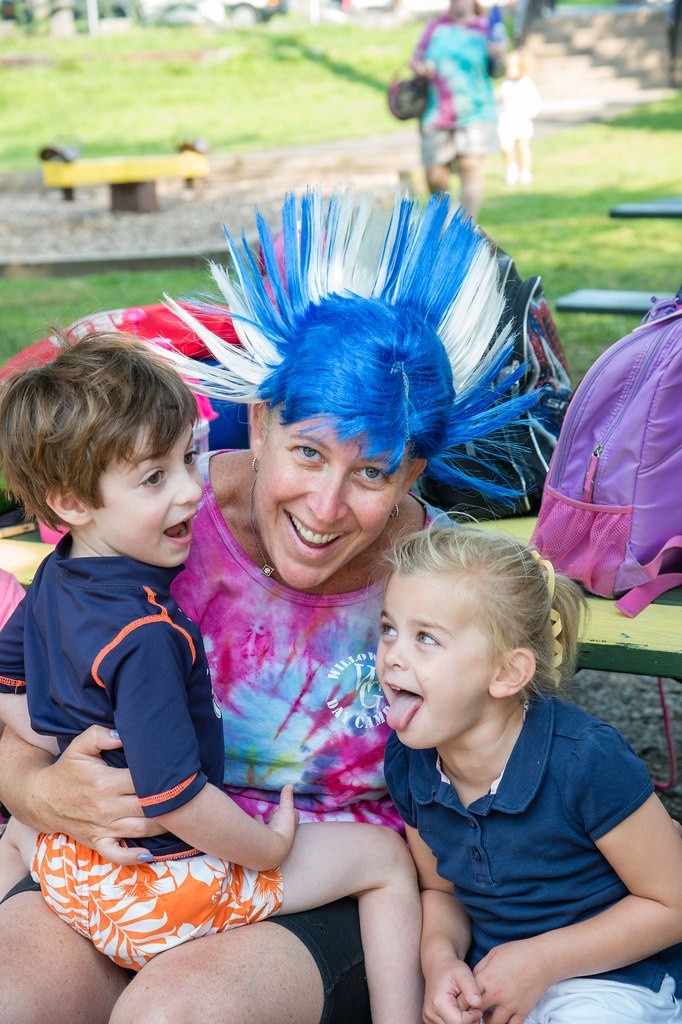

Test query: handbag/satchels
[388,64,425,118]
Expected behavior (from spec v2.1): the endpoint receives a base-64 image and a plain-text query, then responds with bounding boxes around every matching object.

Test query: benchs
[0,512,682,795]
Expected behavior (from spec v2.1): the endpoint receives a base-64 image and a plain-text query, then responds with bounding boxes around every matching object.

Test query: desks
[556,289,675,318]
[609,199,682,218]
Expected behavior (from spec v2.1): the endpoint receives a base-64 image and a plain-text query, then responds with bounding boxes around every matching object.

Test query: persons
[375,526,682,1024]
[497,52,538,185]
[409,0,506,222]
[0,192,551,1024]
[0,330,423,1024]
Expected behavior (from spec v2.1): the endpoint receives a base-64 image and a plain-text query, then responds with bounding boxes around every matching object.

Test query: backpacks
[527,298,681,617]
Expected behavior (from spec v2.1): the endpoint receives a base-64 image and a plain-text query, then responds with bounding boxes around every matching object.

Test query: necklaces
[249,471,276,577]
